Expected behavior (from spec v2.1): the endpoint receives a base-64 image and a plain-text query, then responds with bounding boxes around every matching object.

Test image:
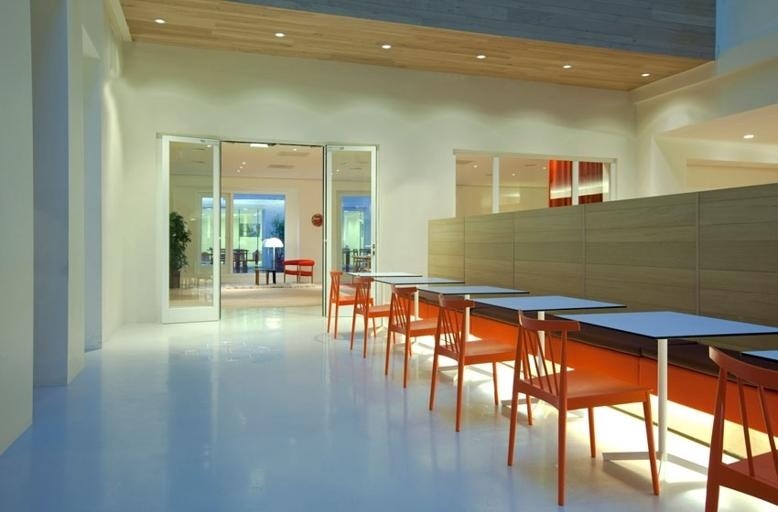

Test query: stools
[254,267,277,285]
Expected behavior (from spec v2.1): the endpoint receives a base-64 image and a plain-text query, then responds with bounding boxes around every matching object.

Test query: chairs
[327,270,379,339]
[349,277,414,359]
[505,309,662,507]
[704,343,776,512]
[427,294,534,433]
[383,286,465,389]
[283,259,315,284]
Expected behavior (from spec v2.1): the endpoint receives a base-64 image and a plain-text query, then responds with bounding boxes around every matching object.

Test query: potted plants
[168,211,191,288]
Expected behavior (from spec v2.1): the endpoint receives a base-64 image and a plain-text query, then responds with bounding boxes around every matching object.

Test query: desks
[465,294,629,424]
[347,271,425,337]
[554,308,776,462]
[372,276,465,348]
[415,284,531,386]
